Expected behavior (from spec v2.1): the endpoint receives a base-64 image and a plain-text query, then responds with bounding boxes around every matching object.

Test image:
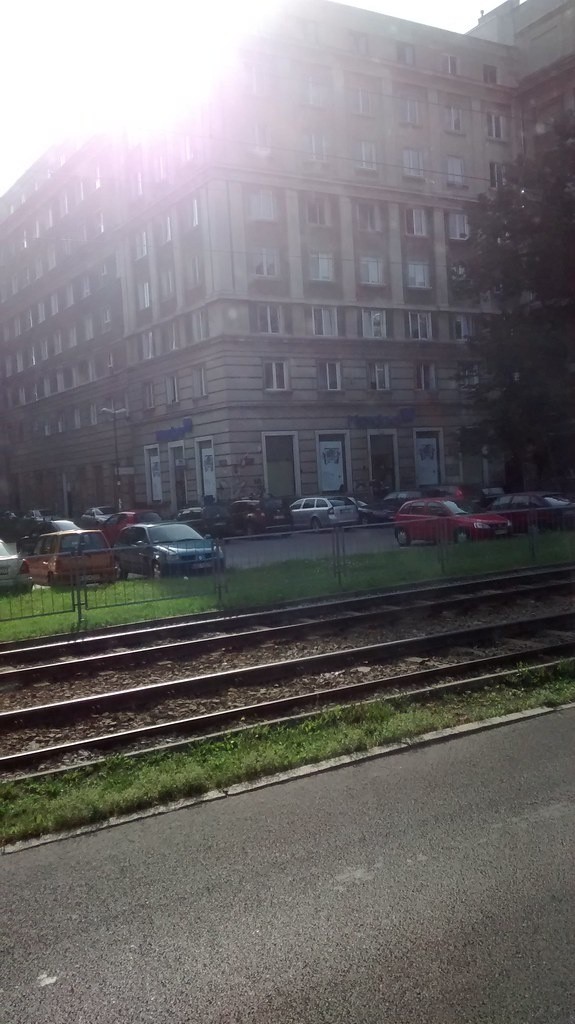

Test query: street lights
[99,406,128,511]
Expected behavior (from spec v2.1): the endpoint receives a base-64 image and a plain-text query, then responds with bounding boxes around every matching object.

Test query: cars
[98,509,164,548]
[481,487,507,502]
[487,488,575,534]
[347,493,402,524]
[22,528,119,587]
[15,520,92,555]
[0,539,35,593]
[76,505,117,531]
[284,495,363,534]
[382,482,488,520]
[0,508,63,543]
[208,493,295,540]
[161,502,227,539]
[113,519,227,584]
[392,496,514,546]
